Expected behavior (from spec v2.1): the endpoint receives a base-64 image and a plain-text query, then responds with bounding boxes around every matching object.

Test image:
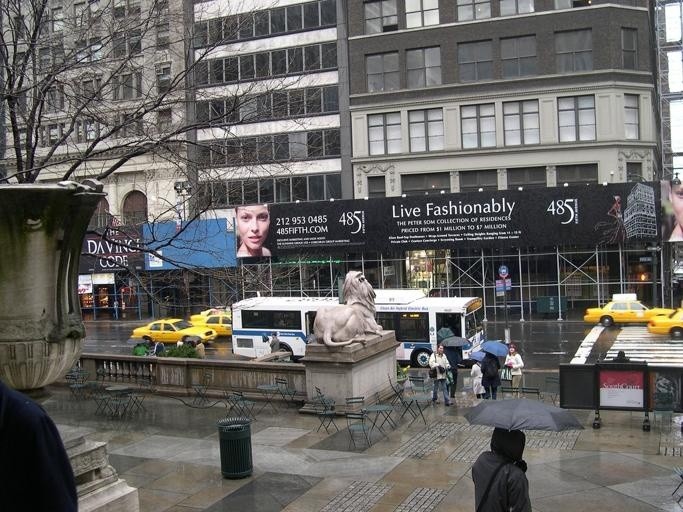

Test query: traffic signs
[495,279,511,292]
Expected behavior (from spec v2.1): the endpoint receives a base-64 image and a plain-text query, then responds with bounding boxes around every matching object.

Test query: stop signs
[497,266,508,277]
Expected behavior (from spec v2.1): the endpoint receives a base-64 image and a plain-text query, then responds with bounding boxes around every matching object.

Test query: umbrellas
[184,336,200,343]
[436,327,454,344]
[127,338,145,344]
[462,397,584,435]
[479,340,508,358]
[468,352,486,363]
[262,332,269,343]
[440,336,468,347]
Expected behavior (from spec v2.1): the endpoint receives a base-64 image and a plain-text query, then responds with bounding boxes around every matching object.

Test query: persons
[470,360,486,399]
[151,339,165,356]
[664,180,682,242]
[235,203,273,260]
[443,346,462,399]
[268,332,280,353]
[427,343,454,406]
[131,343,148,356]
[113,299,119,318]
[480,352,501,400]
[606,194,626,243]
[469,428,532,512]
[193,340,207,359]
[503,343,524,399]
[0,380,79,512]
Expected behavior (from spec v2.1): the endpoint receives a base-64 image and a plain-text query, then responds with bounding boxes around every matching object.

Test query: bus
[231,288,488,369]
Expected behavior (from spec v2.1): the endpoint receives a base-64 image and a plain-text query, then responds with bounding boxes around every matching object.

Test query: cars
[583,294,683,340]
[130,306,232,348]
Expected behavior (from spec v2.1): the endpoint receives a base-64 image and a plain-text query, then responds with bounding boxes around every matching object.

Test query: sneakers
[432,399,440,404]
[445,400,454,406]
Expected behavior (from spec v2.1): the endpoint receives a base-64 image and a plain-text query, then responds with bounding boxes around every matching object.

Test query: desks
[257,385,280,414]
[404,396,433,428]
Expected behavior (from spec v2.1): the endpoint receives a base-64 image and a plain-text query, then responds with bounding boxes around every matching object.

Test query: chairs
[65,368,153,418]
[222,383,257,422]
[193,374,213,406]
[275,378,296,408]
[501,376,560,407]
[313,373,417,448]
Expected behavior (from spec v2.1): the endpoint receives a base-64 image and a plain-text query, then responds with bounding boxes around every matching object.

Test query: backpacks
[487,362,498,378]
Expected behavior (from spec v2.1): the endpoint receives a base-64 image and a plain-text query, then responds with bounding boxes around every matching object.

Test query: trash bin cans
[216,416,254,479]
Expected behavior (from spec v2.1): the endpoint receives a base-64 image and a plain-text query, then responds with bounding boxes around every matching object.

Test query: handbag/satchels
[428,368,437,378]
[500,365,512,381]
[446,368,454,385]
